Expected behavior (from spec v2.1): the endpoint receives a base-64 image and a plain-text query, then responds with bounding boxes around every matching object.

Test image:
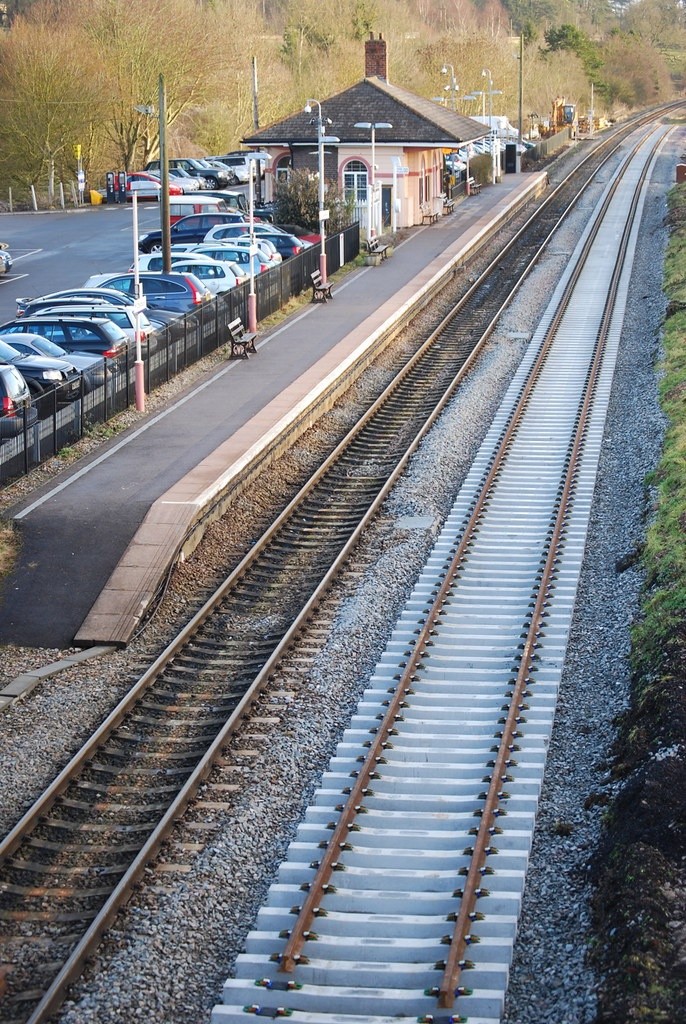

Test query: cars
[110,144,273,201]
[1,200,360,446]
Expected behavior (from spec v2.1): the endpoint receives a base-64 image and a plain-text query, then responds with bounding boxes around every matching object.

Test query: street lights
[305,95,328,284]
[433,60,478,116]
[355,121,394,243]
[473,69,503,154]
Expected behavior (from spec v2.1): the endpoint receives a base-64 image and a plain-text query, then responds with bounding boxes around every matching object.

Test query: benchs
[308,270,333,304]
[439,190,454,214]
[467,176,482,196]
[419,201,439,224]
[366,236,388,262]
[226,318,258,361]
